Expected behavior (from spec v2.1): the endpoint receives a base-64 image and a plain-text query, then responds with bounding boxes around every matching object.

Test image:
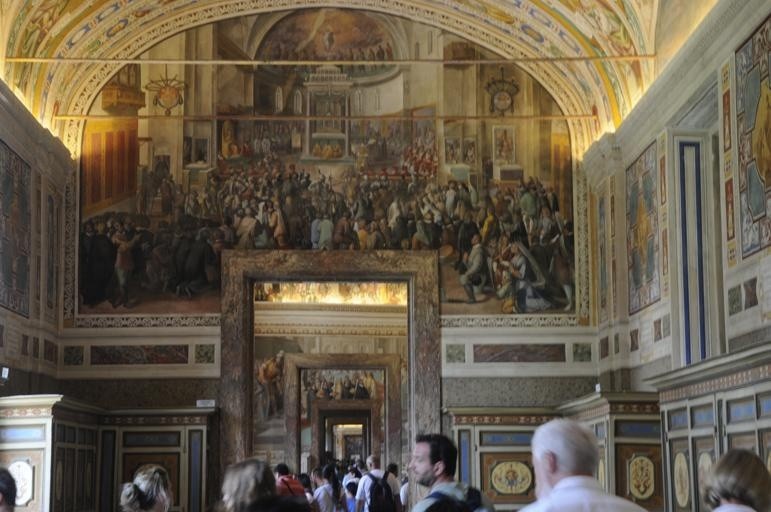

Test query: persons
[258,349,284,418]
[515,415,648,512]
[264,40,393,77]
[299,371,377,421]
[0,465,17,512]
[407,431,496,511]
[700,447,771,511]
[272,448,407,512]
[219,459,315,511]
[119,462,174,512]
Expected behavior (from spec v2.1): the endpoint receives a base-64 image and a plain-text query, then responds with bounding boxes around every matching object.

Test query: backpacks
[365,468,395,512]
